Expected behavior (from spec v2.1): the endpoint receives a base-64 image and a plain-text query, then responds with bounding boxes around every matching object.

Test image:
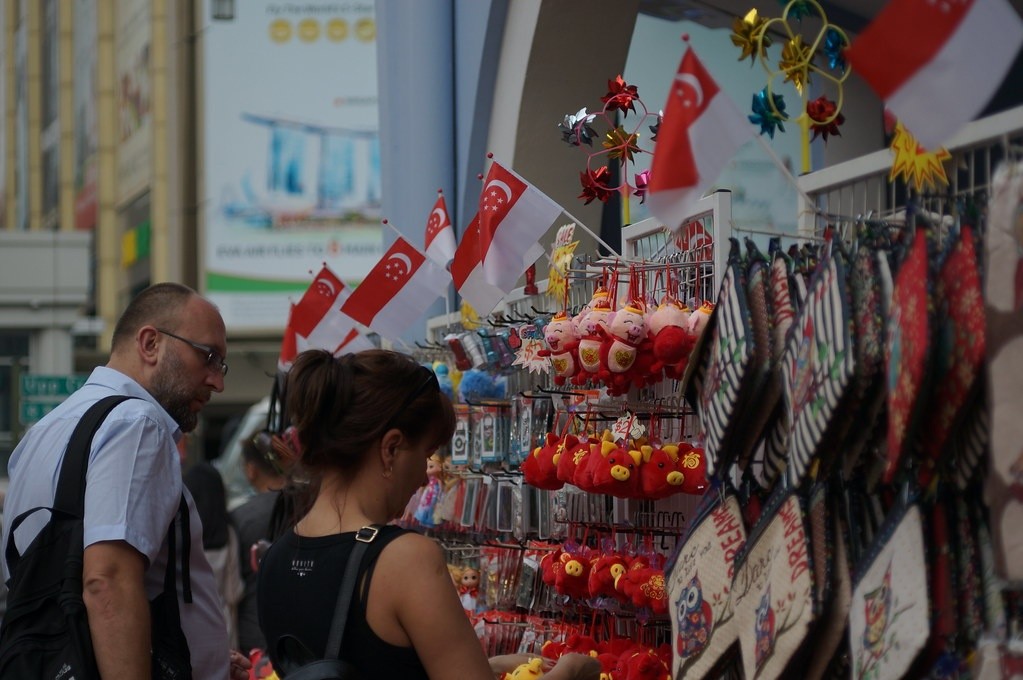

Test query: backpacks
[0,393,191,679]
[272,530,371,679]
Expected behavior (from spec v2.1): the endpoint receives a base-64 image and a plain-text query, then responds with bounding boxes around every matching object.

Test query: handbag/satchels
[666,216,991,678]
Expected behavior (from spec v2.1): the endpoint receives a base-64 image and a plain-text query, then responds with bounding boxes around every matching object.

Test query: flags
[844,0,1023,153]
[646,43,742,234]
[276,161,564,372]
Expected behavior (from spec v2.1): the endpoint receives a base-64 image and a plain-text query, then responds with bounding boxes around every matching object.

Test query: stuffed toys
[502,288,714,679]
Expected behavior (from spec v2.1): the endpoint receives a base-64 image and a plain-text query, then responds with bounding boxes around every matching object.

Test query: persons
[179,464,244,661]
[256,349,603,679]
[0,282,252,680]
[228,425,286,550]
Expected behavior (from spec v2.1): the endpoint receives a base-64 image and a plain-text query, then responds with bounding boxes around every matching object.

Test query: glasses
[158,328,228,377]
